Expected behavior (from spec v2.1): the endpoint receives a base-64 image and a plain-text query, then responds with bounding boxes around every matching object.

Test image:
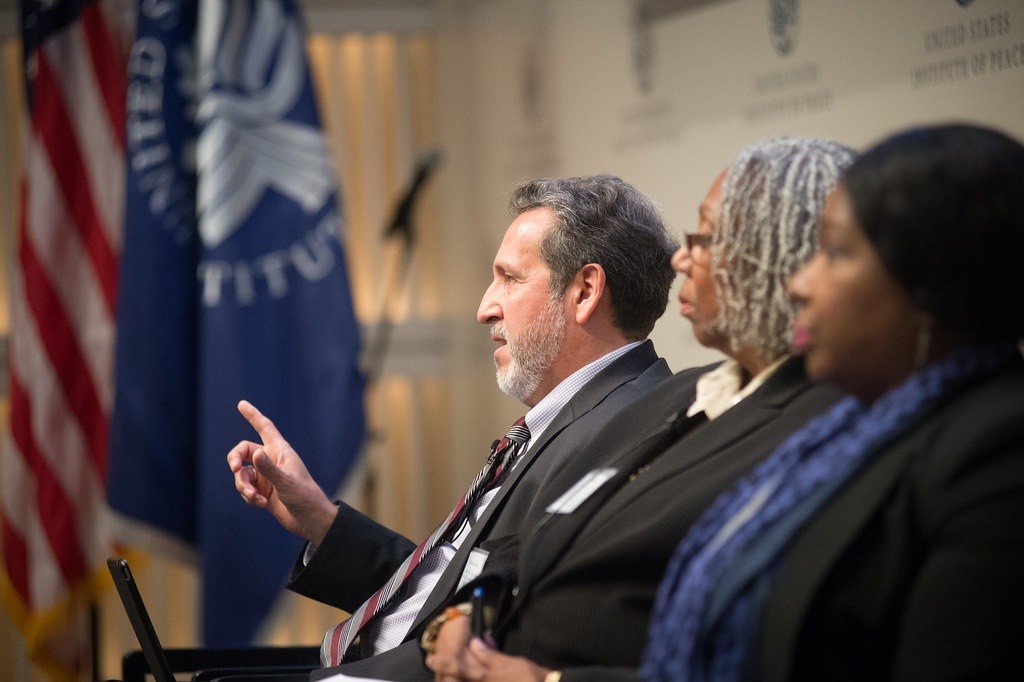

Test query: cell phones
[471,586,485,641]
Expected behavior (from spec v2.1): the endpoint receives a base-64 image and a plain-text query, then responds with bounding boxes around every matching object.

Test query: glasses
[683,228,721,267]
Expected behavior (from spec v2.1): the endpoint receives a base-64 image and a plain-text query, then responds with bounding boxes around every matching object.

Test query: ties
[319,415,531,669]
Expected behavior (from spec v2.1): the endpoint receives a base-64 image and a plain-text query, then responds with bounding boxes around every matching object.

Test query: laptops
[106,556,177,682]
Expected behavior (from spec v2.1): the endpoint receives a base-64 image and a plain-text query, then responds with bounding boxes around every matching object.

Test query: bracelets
[421,601,472,651]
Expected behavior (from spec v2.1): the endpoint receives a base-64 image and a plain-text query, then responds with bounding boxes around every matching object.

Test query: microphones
[488,439,501,457]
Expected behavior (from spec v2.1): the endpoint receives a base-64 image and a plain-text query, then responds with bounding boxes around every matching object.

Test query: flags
[102,0,367,633]
[0,0,133,682]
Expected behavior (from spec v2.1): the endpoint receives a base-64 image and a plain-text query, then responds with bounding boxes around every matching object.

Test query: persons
[420,135,862,682]
[227,175,677,682]
[636,122,1024,682]
